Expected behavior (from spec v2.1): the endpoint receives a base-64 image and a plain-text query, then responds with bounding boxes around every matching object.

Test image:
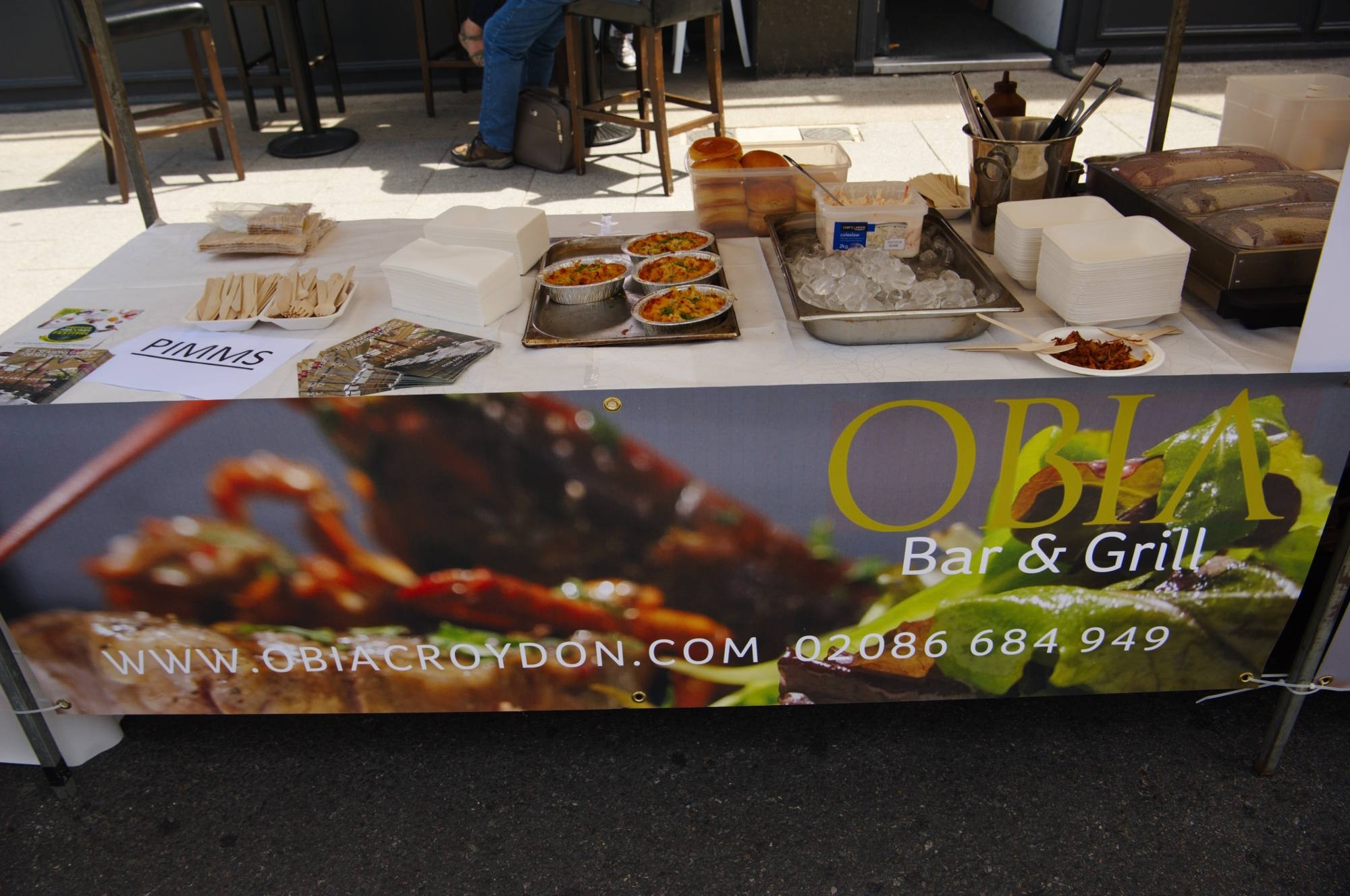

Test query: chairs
[70,0,245,229]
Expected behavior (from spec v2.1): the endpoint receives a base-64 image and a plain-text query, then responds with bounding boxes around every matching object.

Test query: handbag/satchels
[513,85,575,174]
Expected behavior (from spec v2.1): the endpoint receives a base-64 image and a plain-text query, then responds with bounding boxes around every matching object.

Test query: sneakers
[451,130,514,169]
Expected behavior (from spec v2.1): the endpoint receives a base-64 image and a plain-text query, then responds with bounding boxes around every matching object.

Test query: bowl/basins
[631,283,733,336]
[991,196,1191,330]
[181,281,359,330]
[537,255,634,305]
[621,229,716,265]
[630,249,724,296]
[936,185,970,219]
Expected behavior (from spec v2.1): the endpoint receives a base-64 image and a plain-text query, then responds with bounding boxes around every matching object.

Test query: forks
[268,266,356,317]
[944,339,1078,354]
[1095,325,1183,345]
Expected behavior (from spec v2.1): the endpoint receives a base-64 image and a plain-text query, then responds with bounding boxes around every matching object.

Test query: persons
[450,0,637,169]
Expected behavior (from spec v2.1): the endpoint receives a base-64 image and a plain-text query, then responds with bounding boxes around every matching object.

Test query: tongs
[953,71,1005,142]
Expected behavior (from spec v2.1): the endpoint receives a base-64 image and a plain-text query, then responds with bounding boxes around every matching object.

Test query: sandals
[458,24,488,66]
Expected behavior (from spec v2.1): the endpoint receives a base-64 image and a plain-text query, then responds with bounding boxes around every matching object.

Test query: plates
[1032,326,1164,377]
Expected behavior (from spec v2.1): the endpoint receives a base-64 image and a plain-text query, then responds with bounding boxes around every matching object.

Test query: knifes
[196,272,283,320]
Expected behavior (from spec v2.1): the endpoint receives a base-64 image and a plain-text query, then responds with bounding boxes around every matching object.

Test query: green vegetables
[561,236,702,320]
[653,394,1337,698]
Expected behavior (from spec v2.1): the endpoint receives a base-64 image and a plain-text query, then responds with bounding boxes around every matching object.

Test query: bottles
[983,69,1026,117]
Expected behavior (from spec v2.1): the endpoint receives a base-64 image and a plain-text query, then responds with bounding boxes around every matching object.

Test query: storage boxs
[1217,73,1350,171]
[683,140,852,251]
[812,181,927,260]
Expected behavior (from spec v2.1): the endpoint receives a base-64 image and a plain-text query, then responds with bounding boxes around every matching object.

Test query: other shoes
[604,28,637,71]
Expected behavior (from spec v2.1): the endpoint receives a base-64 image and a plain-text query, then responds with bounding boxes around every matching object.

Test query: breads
[690,137,839,233]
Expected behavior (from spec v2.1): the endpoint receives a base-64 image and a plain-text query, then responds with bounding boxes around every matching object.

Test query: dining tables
[0,225,1350,771]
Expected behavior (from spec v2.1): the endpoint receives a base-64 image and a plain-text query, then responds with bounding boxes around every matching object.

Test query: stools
[556,0,726,196]
[223,0,346,132]
[411,0,506,119]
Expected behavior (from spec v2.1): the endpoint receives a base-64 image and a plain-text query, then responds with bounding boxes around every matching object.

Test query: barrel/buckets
[962,117,1083,255]
